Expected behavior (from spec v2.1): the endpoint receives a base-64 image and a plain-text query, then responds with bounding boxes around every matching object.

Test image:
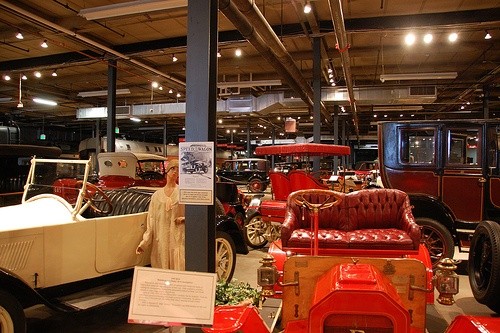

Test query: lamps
[0,0,188,98]
[379,72,458,83]
[372,106,424,112]
[217,80,282,88]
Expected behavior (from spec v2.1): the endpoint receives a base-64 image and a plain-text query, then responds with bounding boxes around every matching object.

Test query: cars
[0,154,250,333]
[374,115,499,304]
[355,161,380,186]
[85,152,168,198]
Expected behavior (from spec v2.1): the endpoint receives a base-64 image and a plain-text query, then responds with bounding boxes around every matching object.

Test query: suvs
[218,158,270,192]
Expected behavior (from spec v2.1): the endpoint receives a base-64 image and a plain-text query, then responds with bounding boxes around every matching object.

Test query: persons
[135,159,186,271]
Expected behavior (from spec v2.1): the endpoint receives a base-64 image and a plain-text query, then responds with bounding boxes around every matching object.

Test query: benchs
[268,169,329,201]
[91,190,155,217]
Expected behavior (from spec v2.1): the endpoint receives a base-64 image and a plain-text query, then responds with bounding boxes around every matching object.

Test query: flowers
[213,277,267,312]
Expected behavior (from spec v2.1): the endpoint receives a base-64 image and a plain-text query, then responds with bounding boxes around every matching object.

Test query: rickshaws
[246,142,352,249]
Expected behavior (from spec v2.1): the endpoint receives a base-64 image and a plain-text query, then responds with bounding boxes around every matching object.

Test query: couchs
[280,187,421,250]
